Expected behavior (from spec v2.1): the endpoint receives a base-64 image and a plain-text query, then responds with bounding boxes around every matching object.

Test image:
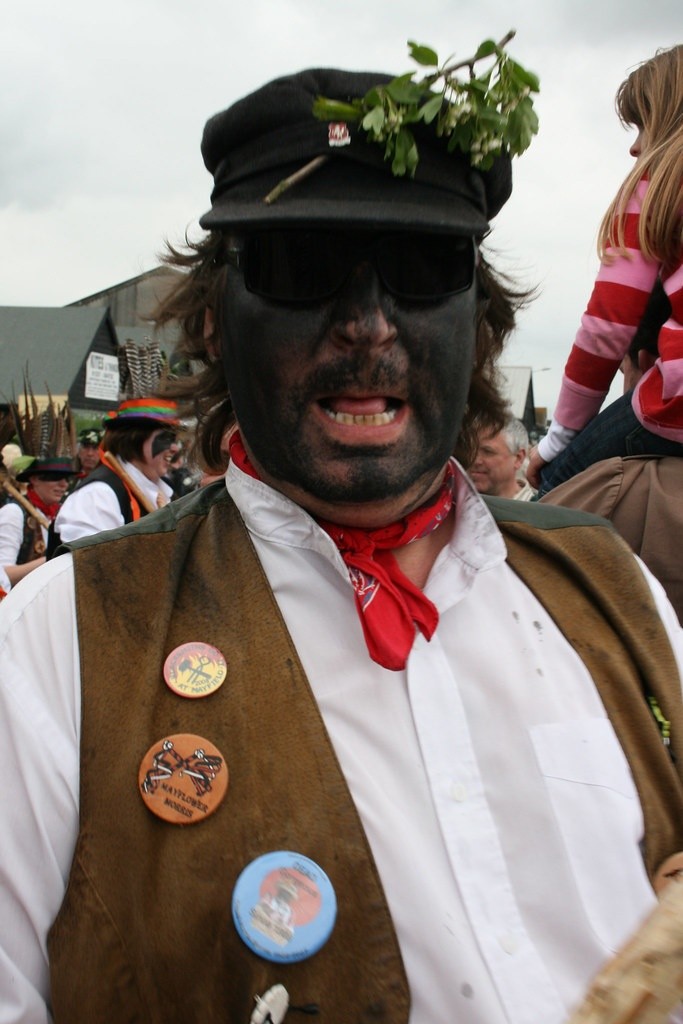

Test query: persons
[0,44,682,594]
[261,876,297,932]
[1,68,683,1024]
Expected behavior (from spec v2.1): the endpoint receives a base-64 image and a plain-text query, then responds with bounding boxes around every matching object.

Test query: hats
[73,412,107,448]
[101,341,182,431]
[199,67,514,238]
[1,364,81,482]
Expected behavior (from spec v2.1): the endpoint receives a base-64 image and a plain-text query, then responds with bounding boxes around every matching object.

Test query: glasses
[221,227,482,304]
